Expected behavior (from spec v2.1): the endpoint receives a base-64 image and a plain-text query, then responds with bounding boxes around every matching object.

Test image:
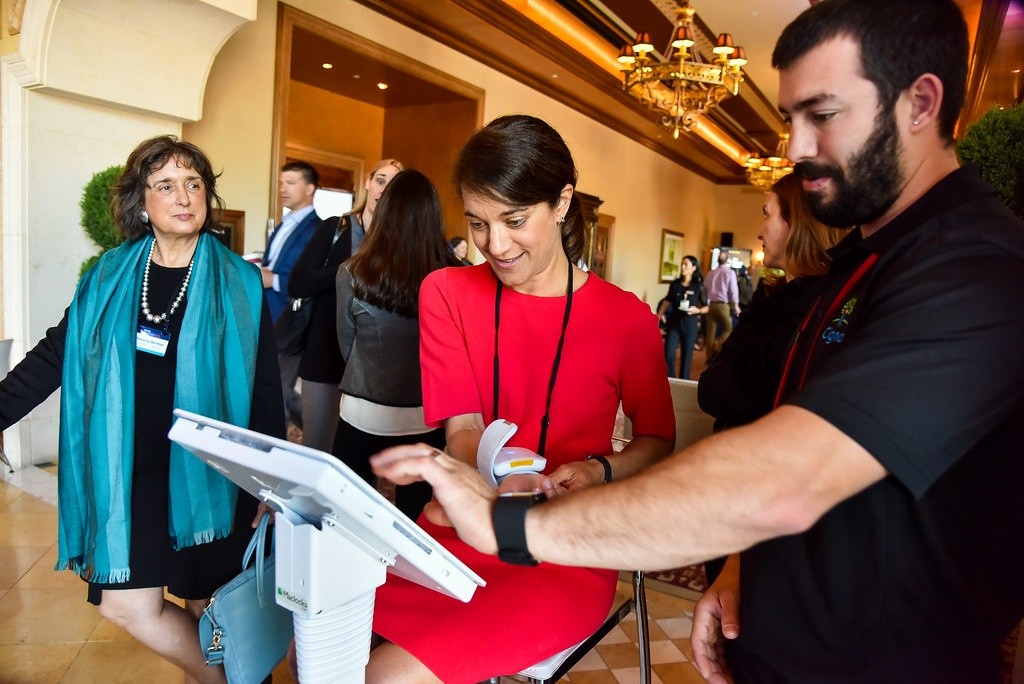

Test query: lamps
[743,122,797,193]
[617,0,747,136]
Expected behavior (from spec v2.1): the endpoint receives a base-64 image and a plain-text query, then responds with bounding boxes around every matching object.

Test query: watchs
[489,489,548,566]
[584,453,613,484]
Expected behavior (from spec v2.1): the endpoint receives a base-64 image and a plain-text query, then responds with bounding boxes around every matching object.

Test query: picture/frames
[658,228,685,283]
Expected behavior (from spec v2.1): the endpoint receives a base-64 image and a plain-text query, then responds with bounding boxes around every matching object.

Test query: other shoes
[261,673,273,684]
[694,343,703,351]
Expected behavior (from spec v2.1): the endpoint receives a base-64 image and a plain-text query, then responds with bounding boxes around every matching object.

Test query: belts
[711,301,724,303]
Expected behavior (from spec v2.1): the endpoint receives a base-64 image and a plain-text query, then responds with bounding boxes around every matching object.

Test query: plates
[679,307,692,312]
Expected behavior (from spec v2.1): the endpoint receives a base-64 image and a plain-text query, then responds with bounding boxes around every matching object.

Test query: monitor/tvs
[165,405,486,604]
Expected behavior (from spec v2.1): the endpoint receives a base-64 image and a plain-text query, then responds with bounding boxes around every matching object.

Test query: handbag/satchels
[198,512,294,684]
[274,297,315,357]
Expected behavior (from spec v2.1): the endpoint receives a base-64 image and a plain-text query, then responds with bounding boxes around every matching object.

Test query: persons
[0,137,289,684]
[260,158,475,523]
[696,172,849,587]
[369,0,1024,684]
[658,252,766,385]
[364,114,676,684]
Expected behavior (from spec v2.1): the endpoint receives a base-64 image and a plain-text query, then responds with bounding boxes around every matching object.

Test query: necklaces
[141,238,194,323]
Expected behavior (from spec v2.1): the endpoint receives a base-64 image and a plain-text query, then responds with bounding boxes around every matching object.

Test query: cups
[680,300,690,309]
